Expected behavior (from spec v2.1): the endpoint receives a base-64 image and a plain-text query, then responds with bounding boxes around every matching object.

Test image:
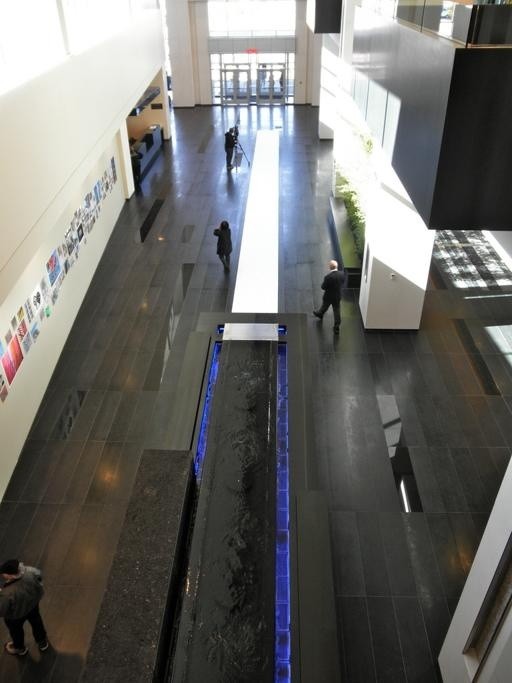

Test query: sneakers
[39,633,48,651]
[5,641,28,656]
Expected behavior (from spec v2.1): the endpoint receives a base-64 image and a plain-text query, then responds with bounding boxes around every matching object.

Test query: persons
[0,559,51,657]
[213,221,231,273]
[223,127,238,169]
[313,258,344,331]
[130,144,143,200]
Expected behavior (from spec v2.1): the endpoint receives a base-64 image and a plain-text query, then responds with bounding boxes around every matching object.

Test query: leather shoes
[333,327,339,334]
[313,310,323,319]
